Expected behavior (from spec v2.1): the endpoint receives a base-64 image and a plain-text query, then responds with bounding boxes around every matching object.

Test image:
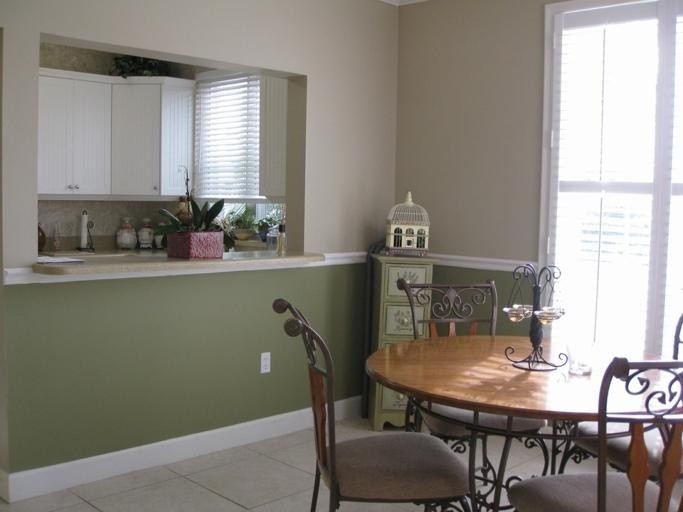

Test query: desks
[363,333,682,511]
[32,244,327,275]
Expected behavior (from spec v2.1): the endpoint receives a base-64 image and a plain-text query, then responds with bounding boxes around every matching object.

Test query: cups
[47,222,65,252]
[566,343,595,376]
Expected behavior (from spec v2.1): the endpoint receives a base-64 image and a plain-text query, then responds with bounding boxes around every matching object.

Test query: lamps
[494,261,569,376]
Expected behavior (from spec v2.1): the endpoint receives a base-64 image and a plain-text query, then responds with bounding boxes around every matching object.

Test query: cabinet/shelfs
[36,65,111,205]
[366,250,442,435]
[112,73,194,206]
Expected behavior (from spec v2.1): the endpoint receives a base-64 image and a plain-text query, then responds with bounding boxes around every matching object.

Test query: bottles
[276,223,287,256]
[38,225,45,251]
[114,218,165,250]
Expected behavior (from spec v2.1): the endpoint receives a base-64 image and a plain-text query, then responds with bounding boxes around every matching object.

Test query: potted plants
[227,204,257,241]
[144,165,234,262]
[251,210,282,243]
[108,54,159,80]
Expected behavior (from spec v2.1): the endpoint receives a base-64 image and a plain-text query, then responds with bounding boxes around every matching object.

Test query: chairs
[555,313,683,482]
[271,297,476,511]
[498,352,682,511]
[382,277,551,488]
